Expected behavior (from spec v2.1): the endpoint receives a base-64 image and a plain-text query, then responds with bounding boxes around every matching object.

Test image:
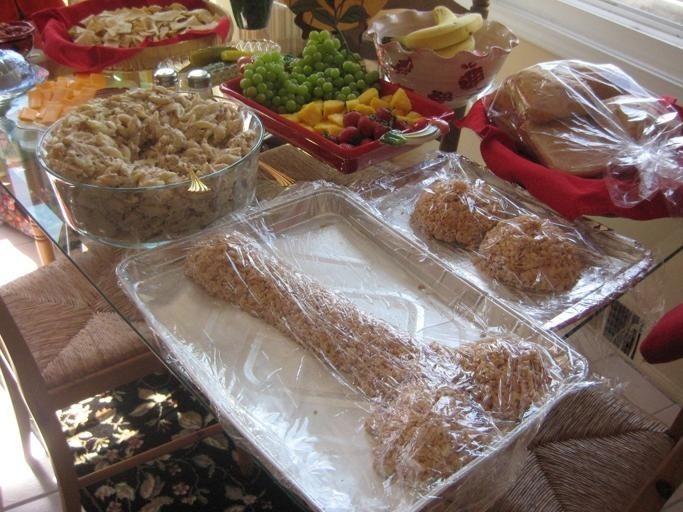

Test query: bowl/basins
[362,8,520,109]
[35,88,264,250]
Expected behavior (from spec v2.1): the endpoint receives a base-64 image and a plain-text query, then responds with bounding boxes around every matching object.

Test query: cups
[0,20,36,57]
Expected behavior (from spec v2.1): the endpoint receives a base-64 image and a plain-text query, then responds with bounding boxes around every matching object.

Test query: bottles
[153,67,179,93]
[186,69,212,99]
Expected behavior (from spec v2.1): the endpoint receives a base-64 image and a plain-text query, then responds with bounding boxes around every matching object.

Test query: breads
[476,213,583,290]
[504,61,683,174]
[408,176,506,243]
[184,226,555,492]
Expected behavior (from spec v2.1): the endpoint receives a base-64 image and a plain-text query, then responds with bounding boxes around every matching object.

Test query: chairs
[425,377,682,512]
[0,252,251,511]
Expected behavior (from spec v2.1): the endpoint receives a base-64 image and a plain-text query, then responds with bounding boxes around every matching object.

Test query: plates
[5,75,140,133]
[156,39,281,97]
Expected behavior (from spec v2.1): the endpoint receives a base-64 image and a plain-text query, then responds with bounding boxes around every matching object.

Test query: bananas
[382,6,483,58]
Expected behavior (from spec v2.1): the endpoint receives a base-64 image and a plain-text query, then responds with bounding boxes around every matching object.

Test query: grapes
[238,30,379,114]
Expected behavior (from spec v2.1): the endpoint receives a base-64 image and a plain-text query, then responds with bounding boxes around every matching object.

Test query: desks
[1,38,683,512]
